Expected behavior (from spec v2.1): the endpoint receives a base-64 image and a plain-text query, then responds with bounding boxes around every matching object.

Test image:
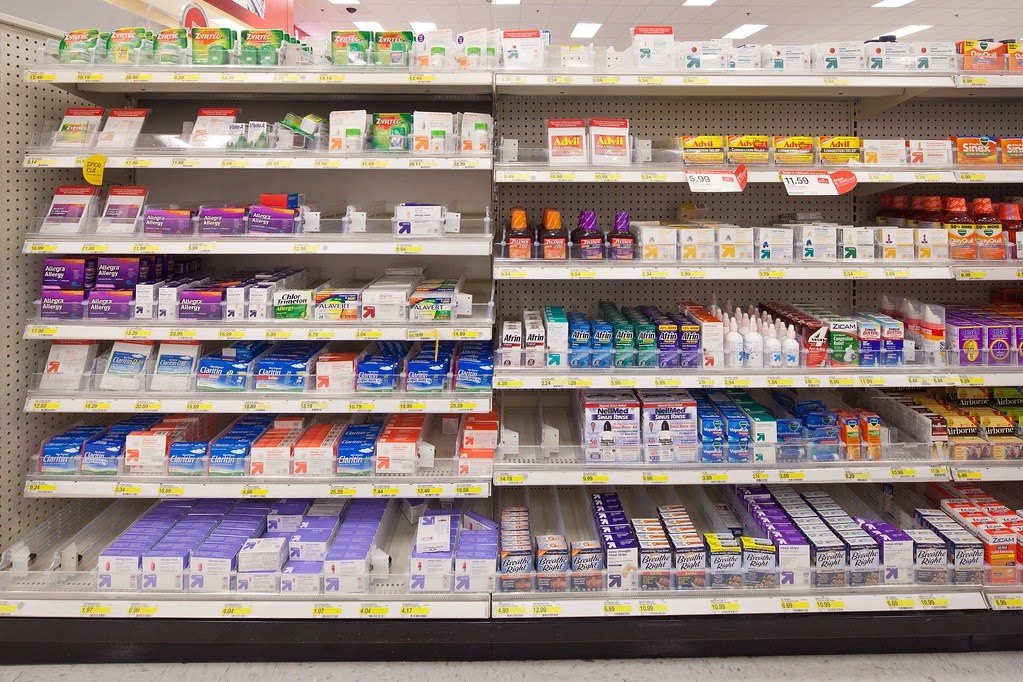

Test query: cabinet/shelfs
[0,19,492,664]
[494,56,972,663]
[856,73,1023,654]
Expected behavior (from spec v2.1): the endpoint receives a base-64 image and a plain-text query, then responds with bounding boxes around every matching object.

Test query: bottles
[873,193,1023,260]
[705,293,800,367]
[572,211,606,259]
[539,207,567,260]
[508,204,532,258]
[607,211,636,260]
[880,294,947,366]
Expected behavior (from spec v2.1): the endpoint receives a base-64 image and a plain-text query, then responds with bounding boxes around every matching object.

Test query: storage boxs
[34,28,1023,593]
